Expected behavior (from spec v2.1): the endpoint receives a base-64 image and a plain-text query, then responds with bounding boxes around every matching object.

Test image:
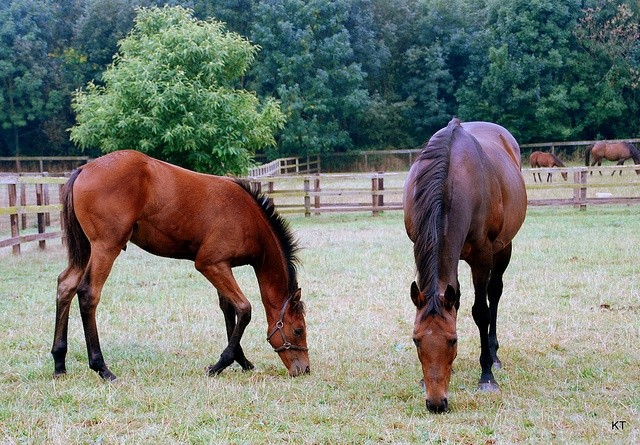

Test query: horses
[530,152,567,183]
[585,141,640,177]
[49,149,313,378]
[403,113,528,415]
